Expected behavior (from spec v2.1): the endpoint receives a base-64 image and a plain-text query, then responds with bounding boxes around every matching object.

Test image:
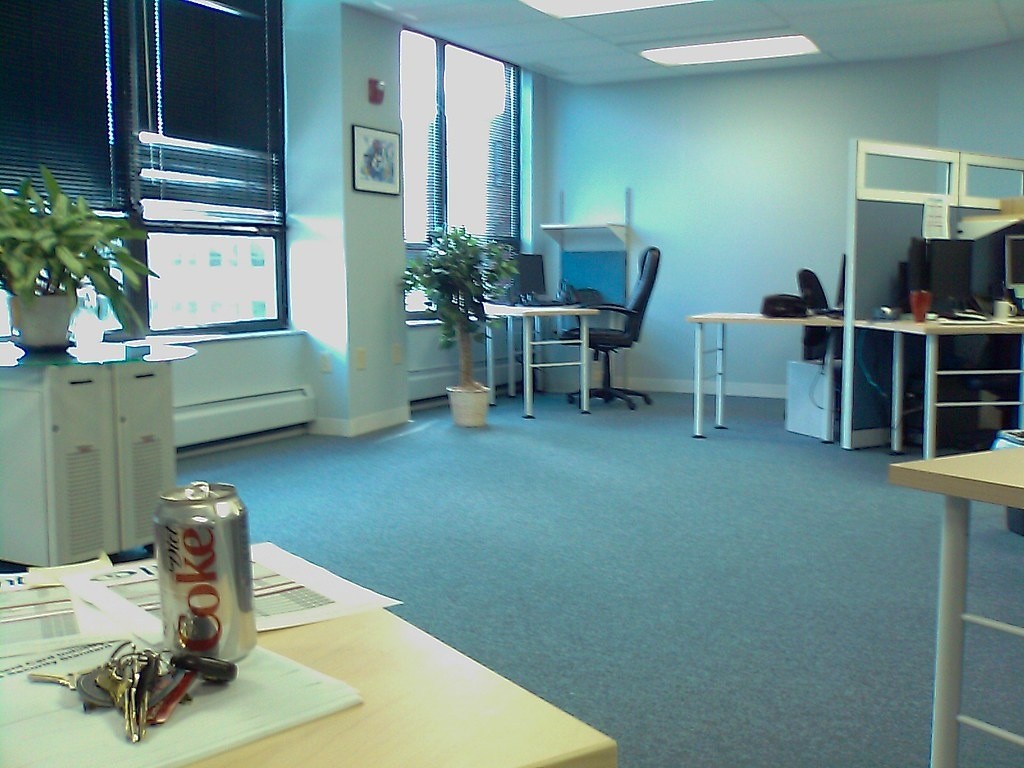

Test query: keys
[27,652,159,742]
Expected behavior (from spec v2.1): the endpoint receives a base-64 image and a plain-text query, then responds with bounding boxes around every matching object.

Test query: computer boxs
[785,360,841,440]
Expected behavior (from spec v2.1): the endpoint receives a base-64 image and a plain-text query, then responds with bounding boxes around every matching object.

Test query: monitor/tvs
[1005,233,1024,289]
[517,253,546,303]
[924,237,974,311]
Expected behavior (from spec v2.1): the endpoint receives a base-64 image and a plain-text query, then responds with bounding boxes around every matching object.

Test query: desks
[687,311,846,442]
[0,542,622,768]
[889,446,1024,768]
[856,309,1024,459]
[481,298,600,417]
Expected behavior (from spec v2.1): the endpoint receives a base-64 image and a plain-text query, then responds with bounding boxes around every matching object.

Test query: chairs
[796,268,842,360]
[558,246,661,410]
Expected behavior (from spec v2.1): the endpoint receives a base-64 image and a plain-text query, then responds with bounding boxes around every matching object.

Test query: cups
[993,301,1016,319]
[911,289,930,321]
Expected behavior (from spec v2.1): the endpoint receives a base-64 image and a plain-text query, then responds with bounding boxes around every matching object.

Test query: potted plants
[0,167,160,349]
[396,220,522,427]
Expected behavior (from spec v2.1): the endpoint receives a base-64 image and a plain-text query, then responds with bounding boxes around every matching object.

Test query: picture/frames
[351,124,401,197]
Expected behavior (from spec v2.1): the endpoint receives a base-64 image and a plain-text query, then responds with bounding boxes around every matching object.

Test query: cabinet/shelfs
[1,338,198,571]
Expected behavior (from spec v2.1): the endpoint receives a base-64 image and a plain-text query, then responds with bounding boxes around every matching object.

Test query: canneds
[150,481,259,663]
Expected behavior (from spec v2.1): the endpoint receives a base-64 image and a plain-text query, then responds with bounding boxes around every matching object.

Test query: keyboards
[530,300,565,307]
